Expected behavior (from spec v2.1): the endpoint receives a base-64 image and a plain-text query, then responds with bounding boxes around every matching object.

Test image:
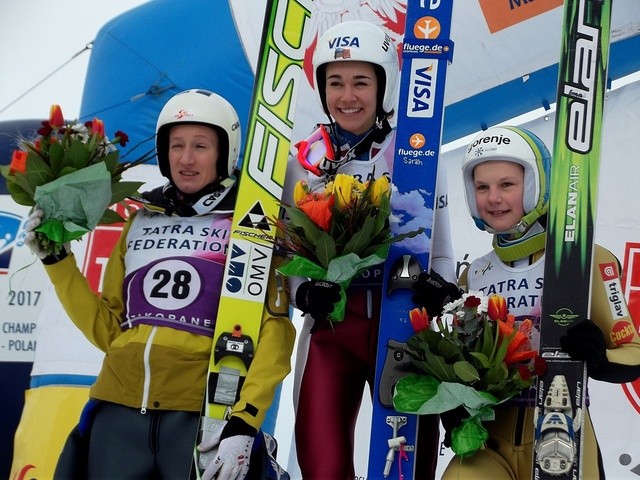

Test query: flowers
[243,173,426,321]
[390,289,549,457]
[0,102,144,252]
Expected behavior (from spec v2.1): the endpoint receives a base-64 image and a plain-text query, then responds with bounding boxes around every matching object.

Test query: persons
[273,22,457,478]
[24,89,295,478]
[441,127,640,478]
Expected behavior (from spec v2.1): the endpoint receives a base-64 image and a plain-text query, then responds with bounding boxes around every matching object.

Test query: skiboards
[532,0,612,480]
[194,0,312,480]
[366,0,455,480]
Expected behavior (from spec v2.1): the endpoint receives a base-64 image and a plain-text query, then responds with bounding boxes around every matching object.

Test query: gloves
[560,318,609,380]
[196,416,258,480]
[295,279,342,320]
[411,271,462,317]
[22,202,71,265]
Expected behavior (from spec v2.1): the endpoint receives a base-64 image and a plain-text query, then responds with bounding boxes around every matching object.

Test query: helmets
[311,20,400,121]
[155,88,241,178]
[462,125,552,235]
